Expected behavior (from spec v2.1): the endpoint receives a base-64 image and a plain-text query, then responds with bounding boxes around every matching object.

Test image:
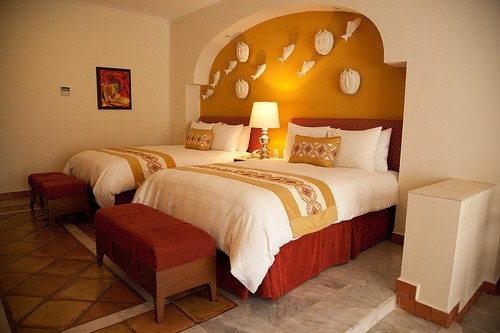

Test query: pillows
[191,122,214,132]
[184,128,213,151]
[374,127,392,173]
[327,126,383,172]
[210,125,244,151]
[222,122,252,151]
[289,135,341,168]
[285,122,329,164]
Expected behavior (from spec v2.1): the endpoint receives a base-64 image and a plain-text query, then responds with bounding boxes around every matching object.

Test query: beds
[62,115,267,209]
[132,118,404,302]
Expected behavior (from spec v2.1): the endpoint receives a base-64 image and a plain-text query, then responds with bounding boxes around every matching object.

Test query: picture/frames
[96,66,133,111]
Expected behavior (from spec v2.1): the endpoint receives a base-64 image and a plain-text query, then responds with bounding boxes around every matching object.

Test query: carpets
[1,197,237,332]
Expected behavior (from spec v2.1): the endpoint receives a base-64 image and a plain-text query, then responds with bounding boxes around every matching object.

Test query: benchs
[27,171,91,230]
[94,204,214,318]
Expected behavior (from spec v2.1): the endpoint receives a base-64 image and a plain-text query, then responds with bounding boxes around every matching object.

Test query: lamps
[249,101,281,160]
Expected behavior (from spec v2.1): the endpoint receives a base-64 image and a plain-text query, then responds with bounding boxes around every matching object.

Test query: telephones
[250,149,261,158]
